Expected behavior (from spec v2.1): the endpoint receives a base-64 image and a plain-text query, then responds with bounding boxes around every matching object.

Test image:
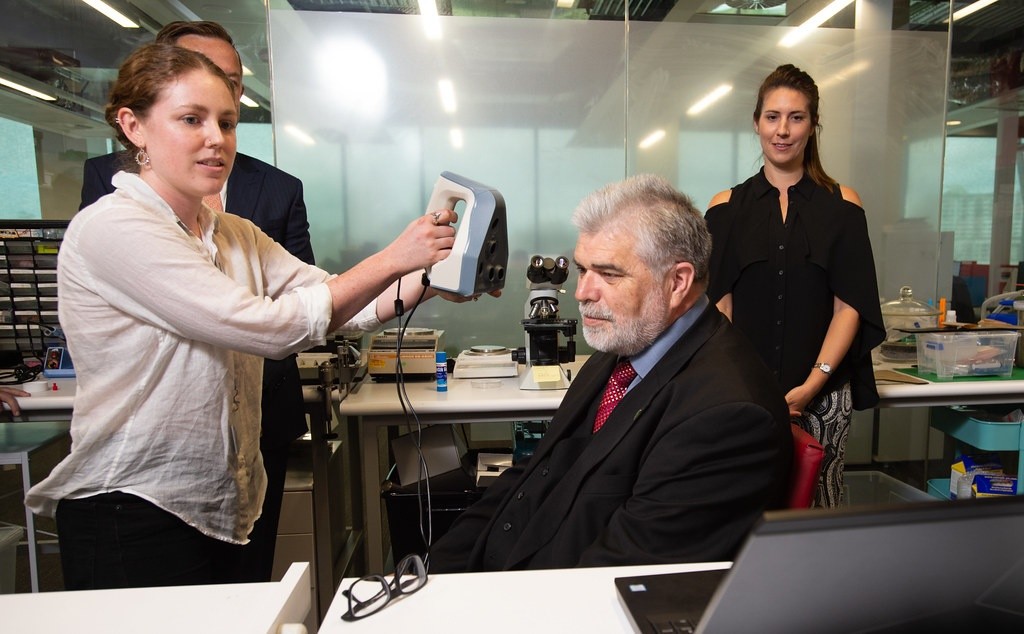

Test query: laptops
[614,493,1024,634]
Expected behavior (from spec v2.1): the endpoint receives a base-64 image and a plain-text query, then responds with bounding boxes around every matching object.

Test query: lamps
[82,0,141,29]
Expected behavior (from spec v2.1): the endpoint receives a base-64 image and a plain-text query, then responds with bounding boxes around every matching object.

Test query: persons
[425,173,798,575]
[22,42,501,591]
[704,64,888,510]
[79,20,315,584]
[0,386,31,416]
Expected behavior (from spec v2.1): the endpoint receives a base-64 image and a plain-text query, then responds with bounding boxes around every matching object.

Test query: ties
[202,192,223,214]
[593,361,638,433]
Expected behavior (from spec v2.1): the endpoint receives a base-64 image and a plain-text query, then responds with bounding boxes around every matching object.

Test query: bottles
[946,310,956,323]
[436,352,447,391]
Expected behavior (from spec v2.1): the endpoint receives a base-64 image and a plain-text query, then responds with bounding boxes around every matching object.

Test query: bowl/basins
[880,286,943,357]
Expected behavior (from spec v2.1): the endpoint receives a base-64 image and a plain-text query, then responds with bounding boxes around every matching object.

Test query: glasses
[341,552,428,622]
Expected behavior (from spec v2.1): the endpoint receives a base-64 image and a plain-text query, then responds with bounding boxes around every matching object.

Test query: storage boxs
[839,470,939,504]
[915,333,1022,379]
[0,525,24,594]
[383,424,514,575]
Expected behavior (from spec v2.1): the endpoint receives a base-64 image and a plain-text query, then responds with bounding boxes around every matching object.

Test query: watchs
[813,361,832,376]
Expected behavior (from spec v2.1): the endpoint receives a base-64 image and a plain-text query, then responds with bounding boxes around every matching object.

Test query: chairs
[784,423,826,508]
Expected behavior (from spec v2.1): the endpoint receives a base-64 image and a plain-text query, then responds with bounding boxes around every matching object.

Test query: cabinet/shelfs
[925,406,1024,501]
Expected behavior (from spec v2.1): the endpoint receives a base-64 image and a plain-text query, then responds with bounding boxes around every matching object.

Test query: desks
[0,560,311,634]
[872,349,1024,475]
[0,369,77,594]
[317,562,738,634]
[340,354,590,575]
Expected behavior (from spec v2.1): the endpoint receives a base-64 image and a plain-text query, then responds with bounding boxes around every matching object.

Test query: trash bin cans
[382,446,515,576]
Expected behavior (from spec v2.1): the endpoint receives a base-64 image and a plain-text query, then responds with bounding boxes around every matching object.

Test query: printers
[881,216,954,303]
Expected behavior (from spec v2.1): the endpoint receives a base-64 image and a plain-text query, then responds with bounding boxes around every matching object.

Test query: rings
[431,211,442,224]
